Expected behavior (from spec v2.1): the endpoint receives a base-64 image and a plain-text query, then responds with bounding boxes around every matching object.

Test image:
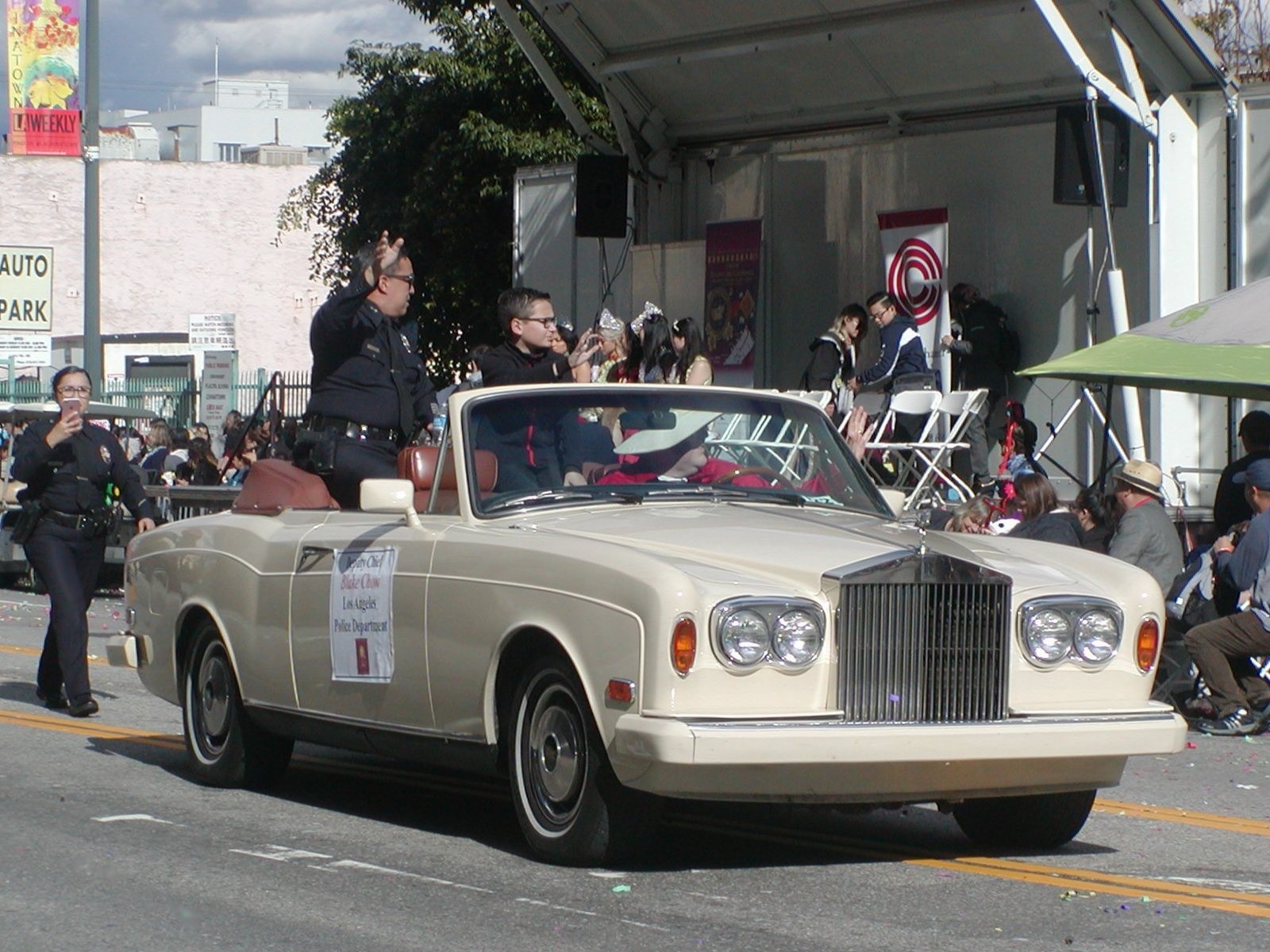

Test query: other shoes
[959,477,995,500]
[68,694,98,716]
[36,684,67,708]
[1253,703,1270,725]
[1197,710,1256,735]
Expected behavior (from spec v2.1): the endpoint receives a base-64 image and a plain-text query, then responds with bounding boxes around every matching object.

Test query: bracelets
[556,357,572,373]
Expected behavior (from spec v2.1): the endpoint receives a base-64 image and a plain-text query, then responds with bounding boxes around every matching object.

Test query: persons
[474,287,606,495]
[591,408,873,498]
[798,303,869,429]
[0,410,305,523]
[580,308,628,422]
[667,317,714,431]
[297,231,452,514]
[1212,409,1270,536]
[926,419,1185,610]
[551,314,591,414]
[10,366,156,719]
[600,322,644,434]
[1183,459,1270,736]
[630,300,674,384]
[942,282,1009,500]
[467,344,493,389]
[846,291,931,504]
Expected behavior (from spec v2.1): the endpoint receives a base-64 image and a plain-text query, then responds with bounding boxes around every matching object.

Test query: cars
[106,383,1187,868]
[0,400,158,594]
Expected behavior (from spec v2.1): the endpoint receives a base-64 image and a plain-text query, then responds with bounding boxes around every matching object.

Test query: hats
[1238,410,1270,437]
[1232,458,1270,489]
[997,420,1037,447]
[1112,459,1165,499]
[613,409,724,455]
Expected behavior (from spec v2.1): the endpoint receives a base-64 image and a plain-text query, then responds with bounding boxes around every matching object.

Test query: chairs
[397,445,498,514]
[1149,594,1215,722]
[703,384,991,511]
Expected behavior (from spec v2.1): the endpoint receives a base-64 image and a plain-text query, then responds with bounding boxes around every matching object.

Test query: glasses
[1068,502,1086,516]
[56,385,92,398]
[386,272,414,290]
[518,316,558,329]
[869,305,891,321]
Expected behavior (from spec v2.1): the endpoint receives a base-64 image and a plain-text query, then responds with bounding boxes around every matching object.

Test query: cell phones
[61,397,81,427]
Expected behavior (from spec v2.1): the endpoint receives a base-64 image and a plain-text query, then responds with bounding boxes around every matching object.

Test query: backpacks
[1164,532,1238,628]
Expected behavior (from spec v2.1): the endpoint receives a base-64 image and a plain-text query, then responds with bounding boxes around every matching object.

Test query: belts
[42,508,86,530]
[304,415,400,443]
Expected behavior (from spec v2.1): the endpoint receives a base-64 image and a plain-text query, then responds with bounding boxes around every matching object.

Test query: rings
[857,430,863,434]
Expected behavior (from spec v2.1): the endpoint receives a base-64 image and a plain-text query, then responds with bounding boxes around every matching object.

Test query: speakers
[574,153,629,239]
[1052,105,1131,208]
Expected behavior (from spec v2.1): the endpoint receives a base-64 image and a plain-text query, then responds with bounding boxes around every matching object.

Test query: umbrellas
[1015,276,1270,402]
